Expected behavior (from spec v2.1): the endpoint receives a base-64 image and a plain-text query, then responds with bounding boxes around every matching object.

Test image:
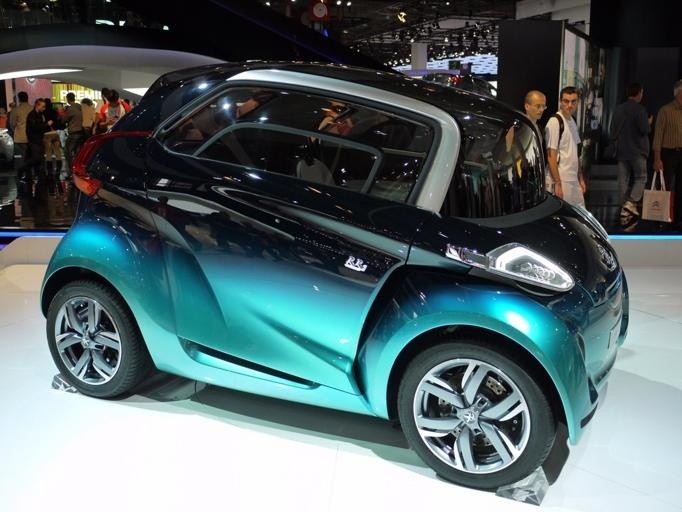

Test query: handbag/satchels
[642,189,673,222]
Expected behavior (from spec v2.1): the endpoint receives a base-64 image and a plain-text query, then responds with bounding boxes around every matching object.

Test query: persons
[541,86,586,207]
[233,90,262,120]
[1,87,132,183]
[293,140,336,186]
[502,89,547,186]
[610,79,653,221]
[320,99,355,137]
[650,79,682,233]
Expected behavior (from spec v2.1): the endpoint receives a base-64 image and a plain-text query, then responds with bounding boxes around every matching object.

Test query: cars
[424,73,497,98]
[39,57,632,489]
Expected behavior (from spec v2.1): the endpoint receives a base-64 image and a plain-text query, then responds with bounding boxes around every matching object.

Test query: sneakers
[621,201,639,217]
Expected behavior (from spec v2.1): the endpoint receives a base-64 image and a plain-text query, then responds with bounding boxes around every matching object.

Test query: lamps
[344,2,509,68]
